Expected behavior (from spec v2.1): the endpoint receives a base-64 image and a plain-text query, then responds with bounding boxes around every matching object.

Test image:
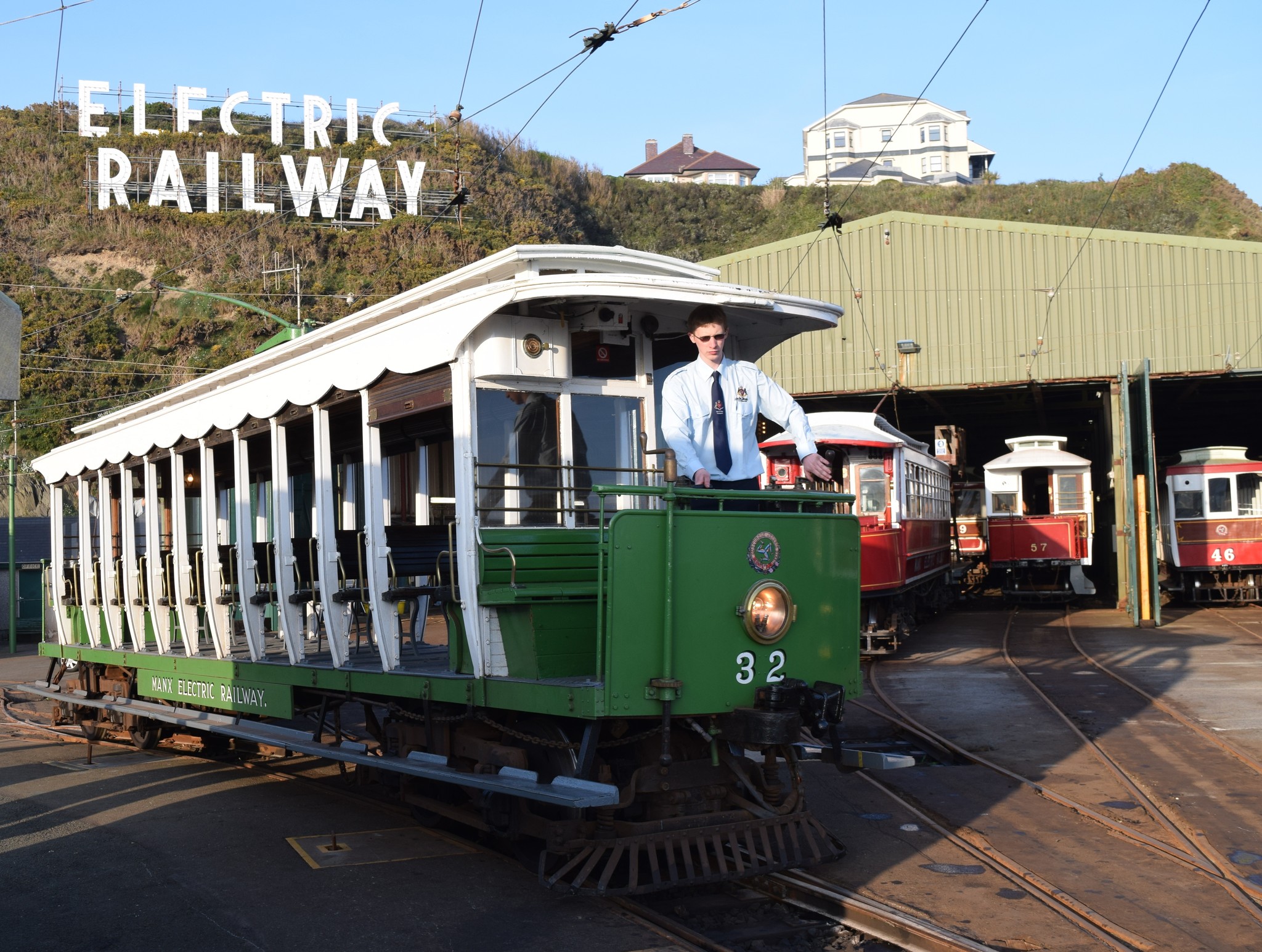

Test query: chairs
[381,524,448,658]
[288,537,322,653]
[110,555,125,606]
[157,550,184,644]
[331,529,380,658]
[215,543,240,648]
[89,558,103,606]
[61,564,81,606]
[133,554,150,606]
[185,548,212,644]
[249,540,287,651]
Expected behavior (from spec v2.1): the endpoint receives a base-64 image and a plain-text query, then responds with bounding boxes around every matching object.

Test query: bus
[1112,445,1262,608]
[757,411,966,663]
[5,243,869,906]
[948,477,995,597]
[982,436,1096,608]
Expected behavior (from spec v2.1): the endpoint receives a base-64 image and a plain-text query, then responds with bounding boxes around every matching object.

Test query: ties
[711,370,732,475]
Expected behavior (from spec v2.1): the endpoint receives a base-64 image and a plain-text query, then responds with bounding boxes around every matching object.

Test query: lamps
[895,339,919,355]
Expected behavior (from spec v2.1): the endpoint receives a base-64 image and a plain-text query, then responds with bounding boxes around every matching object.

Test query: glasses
[693,331,726,342]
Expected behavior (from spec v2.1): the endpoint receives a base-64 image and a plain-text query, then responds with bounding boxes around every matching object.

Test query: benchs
[473,517,609,604]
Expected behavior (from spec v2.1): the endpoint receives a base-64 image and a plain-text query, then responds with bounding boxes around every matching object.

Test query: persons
[480,393,592,527]
[661,306,832,512]
[76,478,161,555]
